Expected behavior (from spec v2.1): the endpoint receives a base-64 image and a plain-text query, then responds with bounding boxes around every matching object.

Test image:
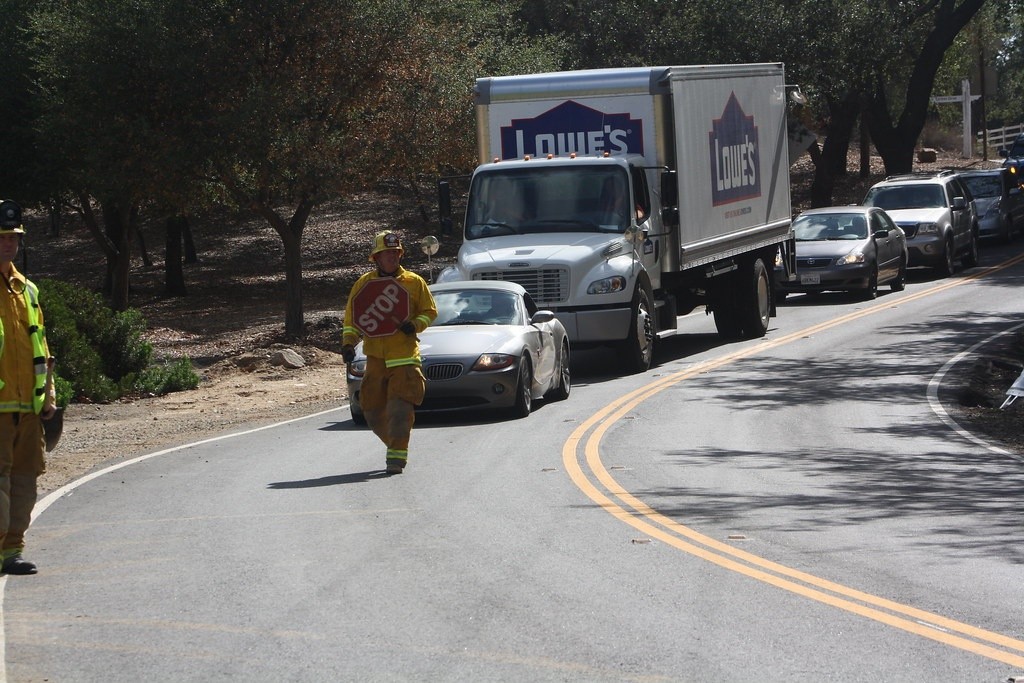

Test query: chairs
[811,220,862,238]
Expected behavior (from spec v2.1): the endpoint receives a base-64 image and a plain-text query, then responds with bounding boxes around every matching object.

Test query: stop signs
[350,279,409,337]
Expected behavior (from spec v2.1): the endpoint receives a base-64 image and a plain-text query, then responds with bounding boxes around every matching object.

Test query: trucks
[419,63,796,374]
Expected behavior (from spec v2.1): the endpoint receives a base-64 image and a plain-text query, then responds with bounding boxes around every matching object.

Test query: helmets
[369,230,404,261]
[0,199,27,236]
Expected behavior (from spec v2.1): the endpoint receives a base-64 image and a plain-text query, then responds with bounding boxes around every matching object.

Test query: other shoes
[386,464,402,473]
[2,555,38,574]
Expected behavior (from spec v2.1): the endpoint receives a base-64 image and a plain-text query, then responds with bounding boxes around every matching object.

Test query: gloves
[399,320,415,334]
[341,344,356,363]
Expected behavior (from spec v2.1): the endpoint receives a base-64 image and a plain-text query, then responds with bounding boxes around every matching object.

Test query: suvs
[860,170,979,277]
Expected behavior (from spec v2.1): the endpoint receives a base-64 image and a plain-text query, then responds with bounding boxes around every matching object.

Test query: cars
[957,168,1024,244]
[775,205,909,300]
[999,132,1024,183]
[346,280,572,425]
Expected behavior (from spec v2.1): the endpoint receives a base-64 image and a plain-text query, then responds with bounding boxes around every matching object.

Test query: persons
[342,230,438,474]
[853,217,873,236]
[0,199,56,573]
[601,177,647,221]
